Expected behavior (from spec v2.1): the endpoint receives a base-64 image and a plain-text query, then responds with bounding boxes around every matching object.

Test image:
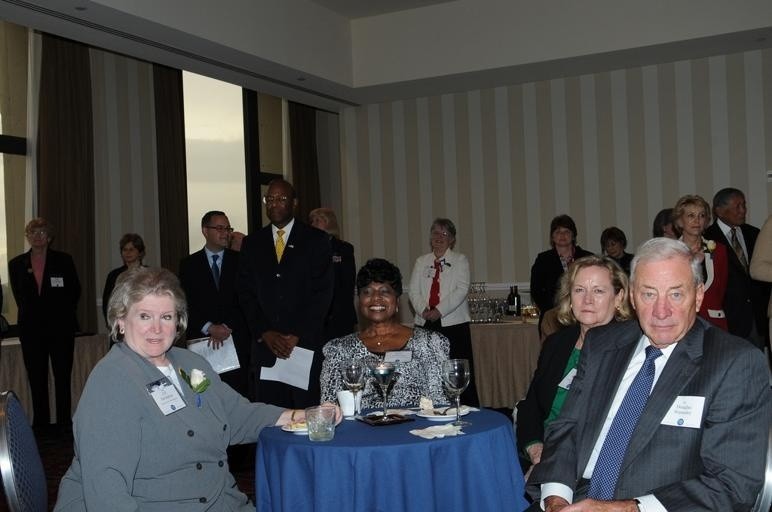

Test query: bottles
[508,286,515,315]
[514,286,521,315]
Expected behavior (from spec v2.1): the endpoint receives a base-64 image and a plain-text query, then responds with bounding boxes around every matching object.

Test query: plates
[416,409,470,422]
[281,424,308,435]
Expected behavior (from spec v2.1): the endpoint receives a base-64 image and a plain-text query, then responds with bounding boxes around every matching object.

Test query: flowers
[179,368,210,409]
[702,239,717,255]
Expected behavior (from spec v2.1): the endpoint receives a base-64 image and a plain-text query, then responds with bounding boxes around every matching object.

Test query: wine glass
[442,359,472,426]
[341,360,367,419]
[367,362,399,422]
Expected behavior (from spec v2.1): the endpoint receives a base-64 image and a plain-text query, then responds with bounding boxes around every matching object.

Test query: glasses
[32,230,48,236]
[430,231,451,238]
[359,285,396,298]
[262,196,291,205]
[205,226,234,233]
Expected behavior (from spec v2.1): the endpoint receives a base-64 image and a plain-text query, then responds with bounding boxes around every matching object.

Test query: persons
[179,176,357,478]
[102,234,149,348]
[516,188,771,512]
[408,218,480,407]
[8,218,82,440]
[54,264,341,512]
[320,259,451,426]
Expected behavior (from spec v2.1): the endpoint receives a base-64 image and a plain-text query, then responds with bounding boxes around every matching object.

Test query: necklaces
[370,330,398,348]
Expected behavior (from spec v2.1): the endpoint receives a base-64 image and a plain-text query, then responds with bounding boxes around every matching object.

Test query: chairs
[751,427,772,512]
[0,390,48,512]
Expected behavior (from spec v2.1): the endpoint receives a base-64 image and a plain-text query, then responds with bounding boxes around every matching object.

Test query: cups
[336,390,363,420]
[305,406,336,442]
[468,280,507,323]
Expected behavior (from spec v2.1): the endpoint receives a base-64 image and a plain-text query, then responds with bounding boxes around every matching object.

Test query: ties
[275,230,286,263]
[730,227,749,277]
[585,345,664,501]
[210,255,219,290]
